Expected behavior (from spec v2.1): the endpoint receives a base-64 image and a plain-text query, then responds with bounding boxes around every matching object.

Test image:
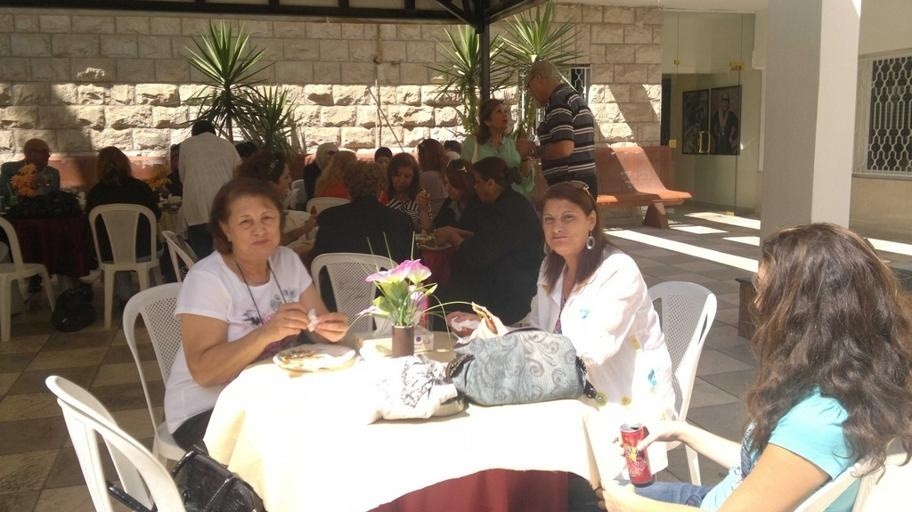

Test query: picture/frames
[682,89,710,155]
[710,86,742,155]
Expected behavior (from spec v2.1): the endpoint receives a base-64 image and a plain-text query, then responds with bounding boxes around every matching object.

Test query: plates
[273,342,356,371]
[412,233,452,251]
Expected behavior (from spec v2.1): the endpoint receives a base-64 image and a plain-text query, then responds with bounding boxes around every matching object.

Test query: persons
[161,174,351,453]
[444,178,675,421]
[595,219,911,511]
[515,59,598,203]
[0,100,544,329]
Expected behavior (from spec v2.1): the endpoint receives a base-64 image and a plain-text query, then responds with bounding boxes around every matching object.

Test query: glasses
[543,179,593,199]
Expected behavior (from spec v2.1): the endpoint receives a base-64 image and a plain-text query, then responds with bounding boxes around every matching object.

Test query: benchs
[532,145,692,228]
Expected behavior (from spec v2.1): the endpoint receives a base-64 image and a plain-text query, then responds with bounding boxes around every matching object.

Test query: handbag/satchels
[51,283,97,333]
[106,442,270,512]
[351,350,468,428]
[442,322,599,410]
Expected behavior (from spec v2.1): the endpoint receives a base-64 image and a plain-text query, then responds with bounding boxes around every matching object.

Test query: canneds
[619,421,654,487]
